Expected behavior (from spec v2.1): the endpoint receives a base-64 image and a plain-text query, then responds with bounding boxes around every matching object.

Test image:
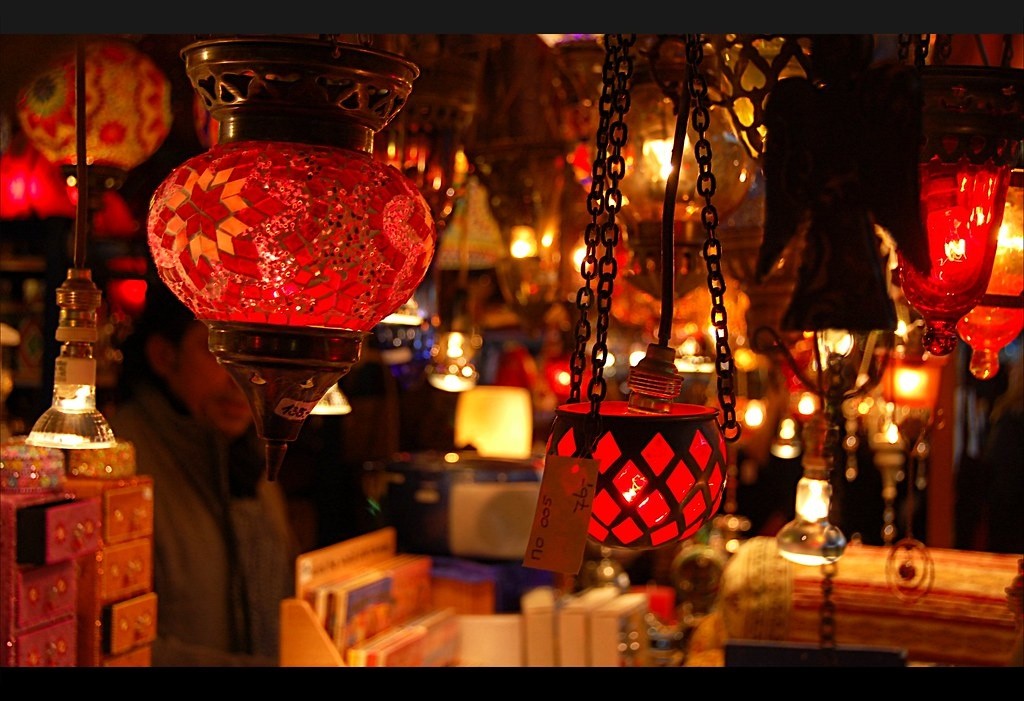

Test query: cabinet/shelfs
[0,474,157,667]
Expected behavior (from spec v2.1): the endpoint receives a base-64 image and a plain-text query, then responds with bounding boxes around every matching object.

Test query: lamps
[894,34,1024,381]
[25,36,116,449]
[147,34,436,479]
[544,34,741,549]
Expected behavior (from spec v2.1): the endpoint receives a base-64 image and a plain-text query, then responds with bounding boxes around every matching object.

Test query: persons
[103,279,300,668]
